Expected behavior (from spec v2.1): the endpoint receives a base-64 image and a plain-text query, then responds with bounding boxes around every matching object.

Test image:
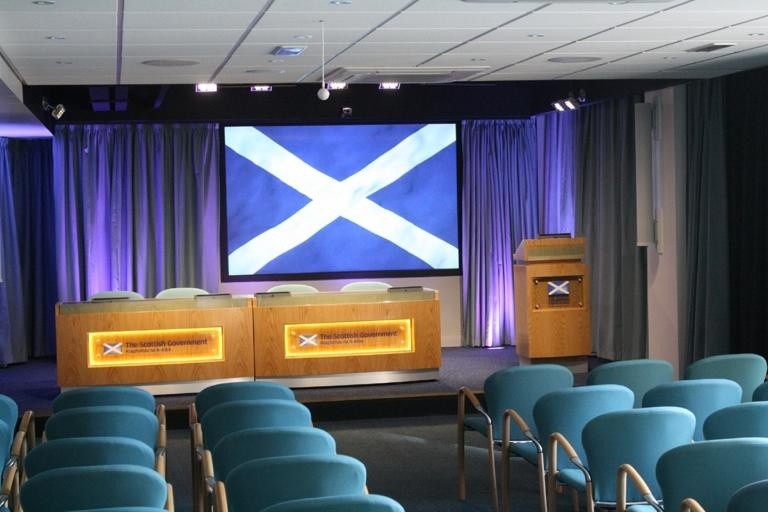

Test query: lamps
[550,90,574,115]
[41,99,66,120]
[563,90,587,115]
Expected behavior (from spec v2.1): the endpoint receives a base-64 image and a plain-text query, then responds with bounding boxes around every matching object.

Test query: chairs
[616,437,768,512]
[188,381,405,512]
[546,406,696,512]
[14,386,175,512]
[501,384,635,512]
[456,363,573,512]
[586,358,674,409]
[641,378,743,443]
[684,353,768,403]
[702,400,768,441]
[0,394,36,512]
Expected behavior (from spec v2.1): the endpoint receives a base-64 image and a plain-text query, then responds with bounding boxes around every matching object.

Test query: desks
[54,298,255,396]
[252,287,442,388]
